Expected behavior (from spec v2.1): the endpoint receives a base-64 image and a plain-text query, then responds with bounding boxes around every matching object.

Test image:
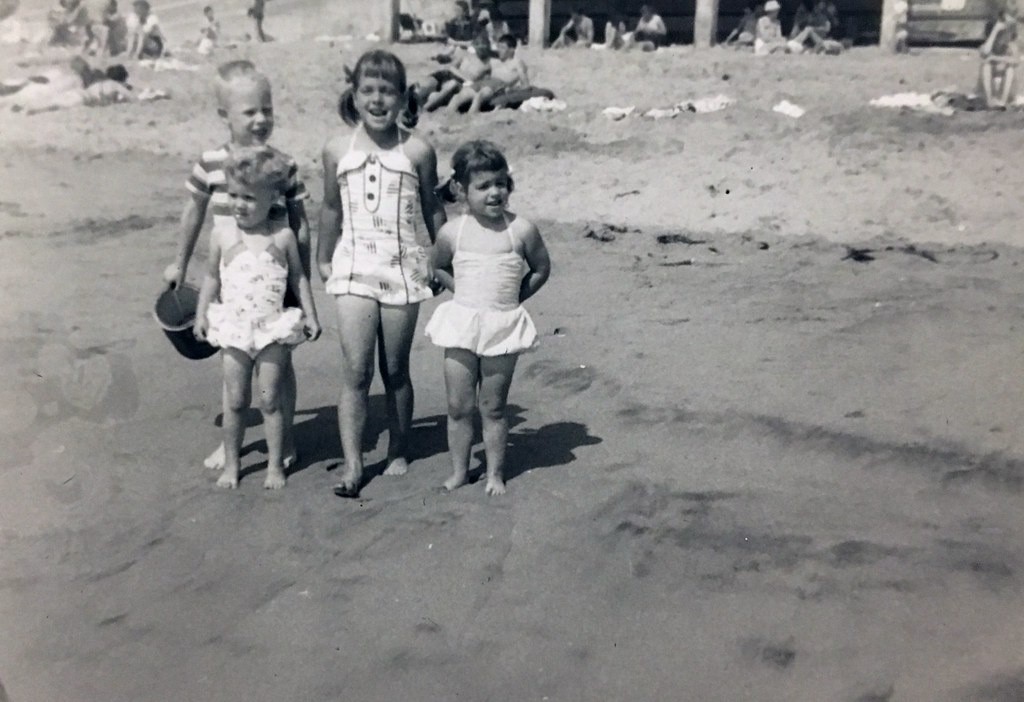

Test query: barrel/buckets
[152,282,221,360]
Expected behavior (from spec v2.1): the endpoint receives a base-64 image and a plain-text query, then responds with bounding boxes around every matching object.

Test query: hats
[763,0,780,12]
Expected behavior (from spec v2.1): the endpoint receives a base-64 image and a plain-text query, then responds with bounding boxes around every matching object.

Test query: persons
[318,50,447,499]
[193,144,315,489]
[17,0,848,115]
[164,61,313,471]
[980,0,1023,110]
[430,140,552,495]
